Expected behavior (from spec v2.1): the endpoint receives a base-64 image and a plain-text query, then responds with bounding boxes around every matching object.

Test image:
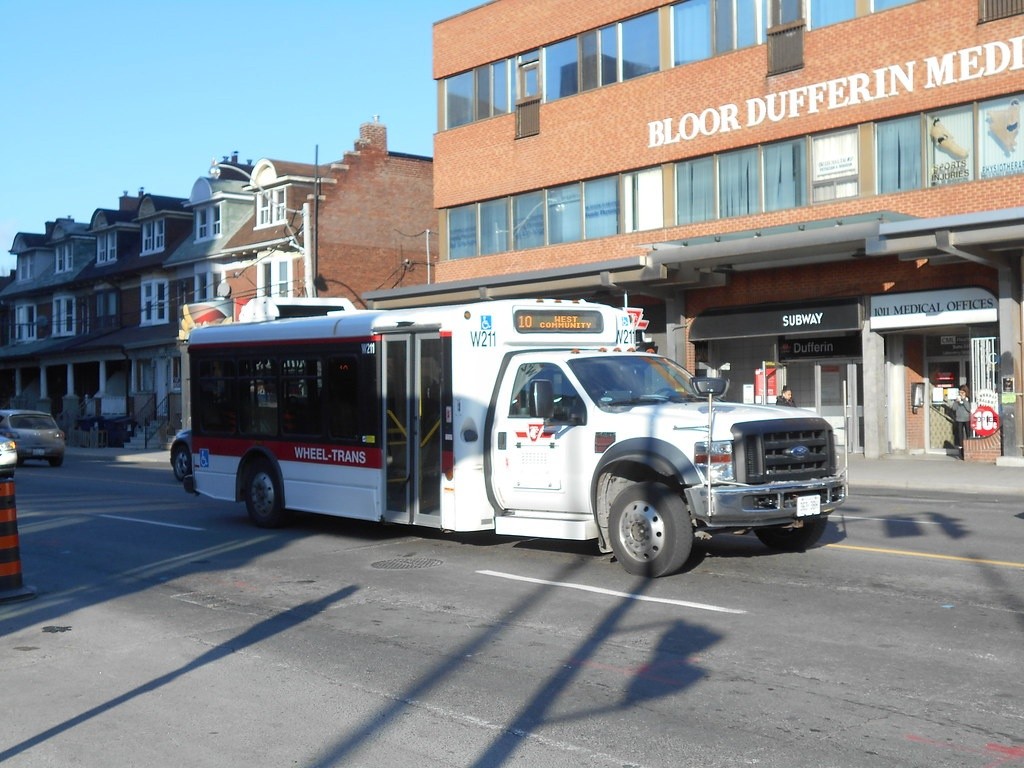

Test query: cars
[0,409,64,467]
[0,435,18,478]
[169,427,193,481]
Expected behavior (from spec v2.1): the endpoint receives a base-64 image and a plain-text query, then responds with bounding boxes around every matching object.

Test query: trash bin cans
[103,415,132,447]
[77,415,105,446]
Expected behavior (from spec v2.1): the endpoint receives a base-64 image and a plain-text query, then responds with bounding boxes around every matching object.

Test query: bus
[184,295,852,579]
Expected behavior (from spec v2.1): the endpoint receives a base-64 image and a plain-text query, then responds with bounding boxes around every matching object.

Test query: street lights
[208,160,314,299]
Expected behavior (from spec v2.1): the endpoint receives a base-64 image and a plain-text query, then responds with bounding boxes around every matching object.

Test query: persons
[776,385,797,408]
[952,385,971,459]
[606,365,640,401]
[192,358,238,435]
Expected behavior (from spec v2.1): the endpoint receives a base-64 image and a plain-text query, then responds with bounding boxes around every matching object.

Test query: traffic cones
[1,477,37,604]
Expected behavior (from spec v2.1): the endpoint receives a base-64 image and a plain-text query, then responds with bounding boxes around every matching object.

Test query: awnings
[686,293,868,344]
